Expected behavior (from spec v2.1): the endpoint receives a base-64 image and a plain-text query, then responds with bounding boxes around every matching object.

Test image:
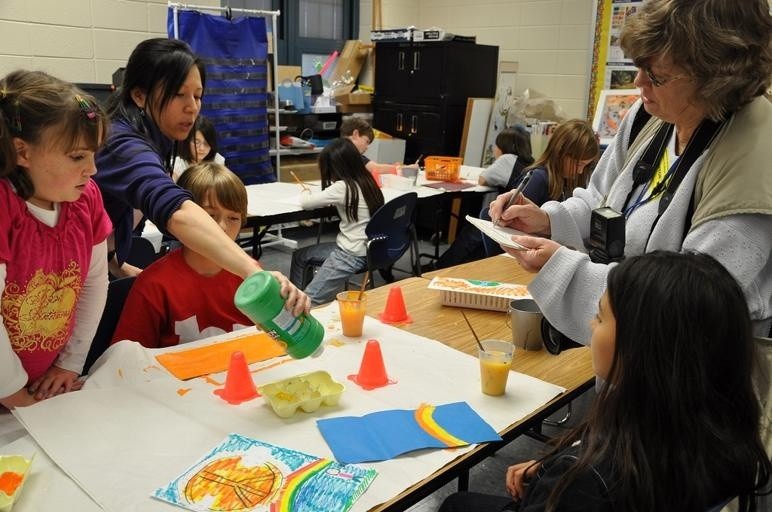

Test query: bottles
[232,271,325,360]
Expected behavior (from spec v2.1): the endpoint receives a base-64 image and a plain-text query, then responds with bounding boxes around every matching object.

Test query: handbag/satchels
[278,75,323,116]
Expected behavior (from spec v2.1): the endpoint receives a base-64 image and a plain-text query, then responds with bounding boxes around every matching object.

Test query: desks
[305,169,498,271]
[158,180,339,264]
[421,247,633,445]
[2,275,597,512]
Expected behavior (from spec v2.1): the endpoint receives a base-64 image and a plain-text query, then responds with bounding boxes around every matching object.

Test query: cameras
[540,206,626,356]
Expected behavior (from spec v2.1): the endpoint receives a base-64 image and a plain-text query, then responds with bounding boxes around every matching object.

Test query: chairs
[479,204,506,258]
[302,191,420,289]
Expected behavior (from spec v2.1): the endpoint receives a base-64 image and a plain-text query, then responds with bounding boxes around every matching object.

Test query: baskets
[425,156,465,181]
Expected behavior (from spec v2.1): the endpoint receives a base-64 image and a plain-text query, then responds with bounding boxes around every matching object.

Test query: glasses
[645,66,697,88]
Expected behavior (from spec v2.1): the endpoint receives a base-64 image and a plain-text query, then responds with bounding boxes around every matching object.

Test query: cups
[477,340,515,395]
[335,291,370,339]
[401,167,418,186]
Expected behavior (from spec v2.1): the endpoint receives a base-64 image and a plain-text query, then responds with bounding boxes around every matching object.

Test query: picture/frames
[592,89,642,146]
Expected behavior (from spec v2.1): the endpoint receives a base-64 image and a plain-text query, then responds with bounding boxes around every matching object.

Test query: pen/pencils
[355,270,370,306]
[290,171,308,190]
[493,170,533,229]
[415,154,423,165]
[460,312,487,355]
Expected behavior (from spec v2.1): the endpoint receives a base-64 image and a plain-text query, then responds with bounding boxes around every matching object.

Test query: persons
[421,125,539,273]
[526,119,602,199]
[340,115,420,175]
[106,162,300,347]
[487,1,771,345]
[296,138,389,304]
[88,38,312,333]
[438,250,771,510]
[168,114,228,187]
[1,69,114,419]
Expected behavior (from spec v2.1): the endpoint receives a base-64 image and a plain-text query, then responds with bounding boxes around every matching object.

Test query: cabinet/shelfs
[267,109,347,139]
[372,104,467,163]
[376,41,500,105]
